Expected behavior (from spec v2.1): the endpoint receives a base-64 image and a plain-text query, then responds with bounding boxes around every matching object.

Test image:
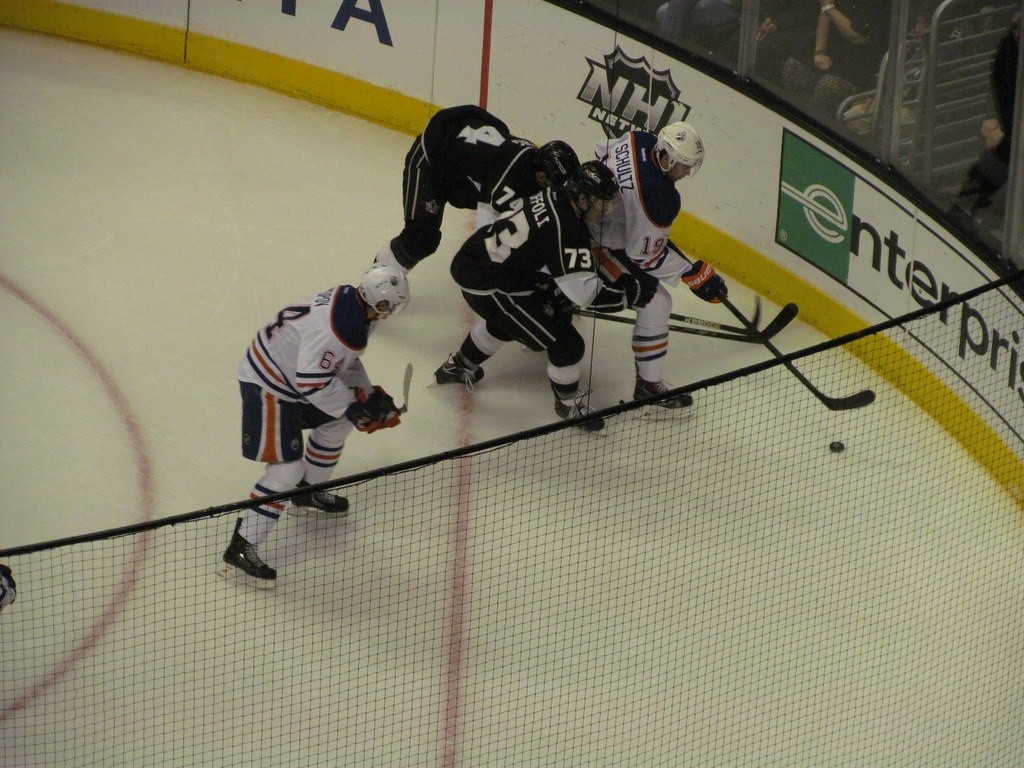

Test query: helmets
[654,121,705,168]
[568,159,620,205]
[358,264,411,316]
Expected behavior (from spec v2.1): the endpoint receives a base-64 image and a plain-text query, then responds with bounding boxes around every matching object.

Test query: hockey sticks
[396,364,414,413]
[670,292,762,335]
[667,238,876,411]
[574,302,800,343]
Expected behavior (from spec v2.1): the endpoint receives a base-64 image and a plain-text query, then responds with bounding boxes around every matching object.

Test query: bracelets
[815,51,825,55]
[821,3,835,12]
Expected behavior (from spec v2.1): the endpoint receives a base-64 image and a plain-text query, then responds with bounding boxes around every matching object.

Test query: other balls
[829,441,844,453]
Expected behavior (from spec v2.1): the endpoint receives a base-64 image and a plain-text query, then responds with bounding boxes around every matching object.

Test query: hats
[534,139,580,188]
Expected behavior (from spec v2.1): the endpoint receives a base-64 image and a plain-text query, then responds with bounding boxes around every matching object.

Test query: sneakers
[286,480,349,518]
[427,353,484,392]
[632,360,692,419]
[217,517,277,589]
[551,384,607,436]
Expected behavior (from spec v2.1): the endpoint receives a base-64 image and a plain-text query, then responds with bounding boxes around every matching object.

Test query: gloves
[681,259,728,303]
[347,385,401,433]
[618,272,658,308]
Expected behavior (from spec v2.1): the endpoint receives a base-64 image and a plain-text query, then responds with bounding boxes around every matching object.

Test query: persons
[655,0,1024,230]
[218,262,411,589]
[374,104,728,437]
